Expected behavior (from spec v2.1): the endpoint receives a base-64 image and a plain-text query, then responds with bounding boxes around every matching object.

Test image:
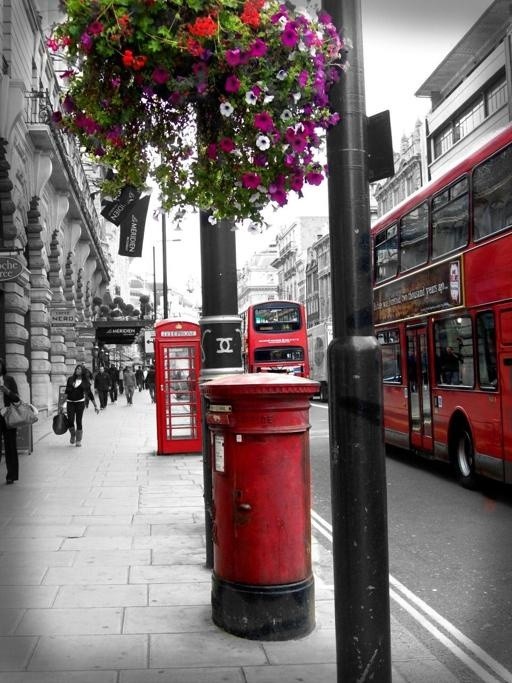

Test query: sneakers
[6,480,13,485]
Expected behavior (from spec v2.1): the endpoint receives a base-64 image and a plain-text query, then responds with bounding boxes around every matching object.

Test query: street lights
[152,238,185,321]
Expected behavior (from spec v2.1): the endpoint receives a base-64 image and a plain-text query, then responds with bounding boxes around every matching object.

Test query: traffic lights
[369,109,394,181]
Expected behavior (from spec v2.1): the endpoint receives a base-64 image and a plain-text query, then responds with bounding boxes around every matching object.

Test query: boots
[75,430,83,446]
[68,427,75,444]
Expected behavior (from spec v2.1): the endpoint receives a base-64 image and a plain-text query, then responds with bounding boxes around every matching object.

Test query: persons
[110,364,156,405]
[80,362,95,409]
[0,359,18,484]
[61,364,99,446]
[94,366,112,410]
[383,340,459,392]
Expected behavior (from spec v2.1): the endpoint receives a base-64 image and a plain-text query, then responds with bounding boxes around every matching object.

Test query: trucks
[307,320,334,402]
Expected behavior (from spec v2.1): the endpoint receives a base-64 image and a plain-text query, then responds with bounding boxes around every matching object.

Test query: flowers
[132,324,154,360]
[41,0,351,232]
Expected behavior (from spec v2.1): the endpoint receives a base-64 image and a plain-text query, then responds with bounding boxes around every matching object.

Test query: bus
[370,123,512,486]
[238,299,310,386]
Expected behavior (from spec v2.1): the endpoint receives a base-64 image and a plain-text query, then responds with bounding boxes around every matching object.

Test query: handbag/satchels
[3,404,38,427]
[53,414,68,434]
